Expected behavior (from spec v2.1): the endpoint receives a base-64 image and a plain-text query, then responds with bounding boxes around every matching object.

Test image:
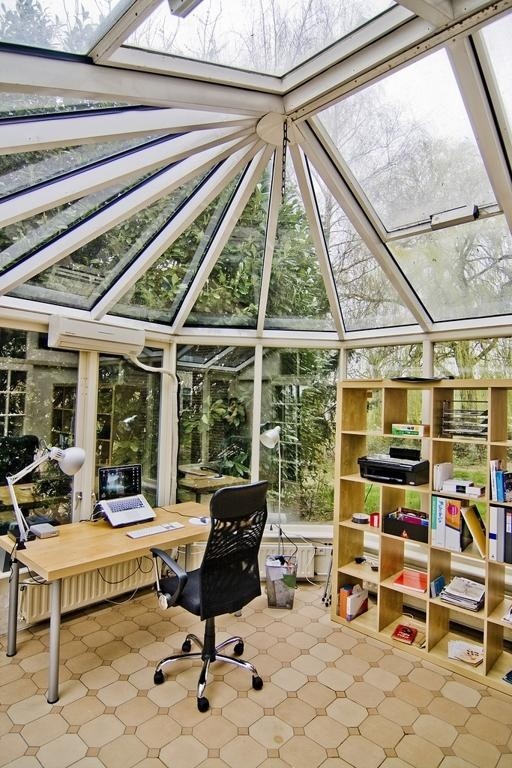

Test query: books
[412,631,426,647]
[430,459,512,685]
[393,570,427,593]
[391,624,418,644]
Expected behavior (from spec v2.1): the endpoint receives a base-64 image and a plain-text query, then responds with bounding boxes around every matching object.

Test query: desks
[177,463,248,503]
[0,483,69,517]
[0,501,211,704]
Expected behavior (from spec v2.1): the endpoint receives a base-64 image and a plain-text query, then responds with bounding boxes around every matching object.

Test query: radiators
[184,542,314,579]
[16,556,162,625]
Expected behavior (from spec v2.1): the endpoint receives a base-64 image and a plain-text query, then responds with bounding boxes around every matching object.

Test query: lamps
[260,426,283,556]
[6,446,85,550]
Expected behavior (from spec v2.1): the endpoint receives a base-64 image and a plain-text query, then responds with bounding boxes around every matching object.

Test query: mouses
[201,518,210,523]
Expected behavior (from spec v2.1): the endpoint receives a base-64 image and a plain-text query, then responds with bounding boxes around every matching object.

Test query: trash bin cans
[265,554,298,610]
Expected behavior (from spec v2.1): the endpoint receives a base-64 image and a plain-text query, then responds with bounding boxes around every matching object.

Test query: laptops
[98,464,156,527]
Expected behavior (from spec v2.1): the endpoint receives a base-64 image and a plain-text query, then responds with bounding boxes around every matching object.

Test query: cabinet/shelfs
[331,379,512,695]
[51,384,113,476]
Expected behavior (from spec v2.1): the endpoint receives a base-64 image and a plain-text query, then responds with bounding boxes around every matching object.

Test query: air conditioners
[48,316,145,357]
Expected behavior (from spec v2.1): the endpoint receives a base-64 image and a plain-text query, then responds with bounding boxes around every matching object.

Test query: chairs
[0,434,39,518]
[149,480,268,712]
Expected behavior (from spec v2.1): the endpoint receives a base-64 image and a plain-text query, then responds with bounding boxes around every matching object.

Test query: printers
[358,448,429,486]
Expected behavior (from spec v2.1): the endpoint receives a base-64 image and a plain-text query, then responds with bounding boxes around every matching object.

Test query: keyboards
[127,521,185,539]
